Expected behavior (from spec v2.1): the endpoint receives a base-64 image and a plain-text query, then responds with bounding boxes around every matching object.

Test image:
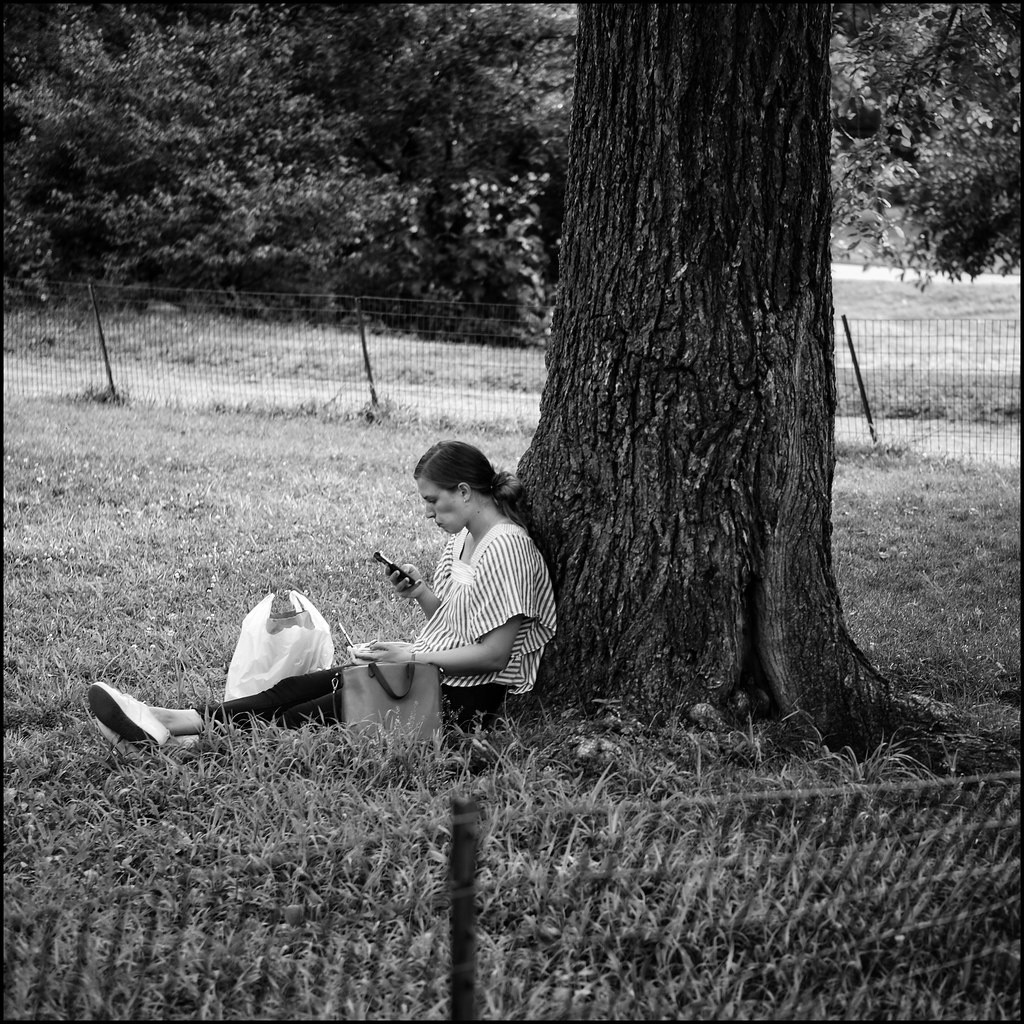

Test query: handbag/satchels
[225,588,333,701]
[341,661,443,745]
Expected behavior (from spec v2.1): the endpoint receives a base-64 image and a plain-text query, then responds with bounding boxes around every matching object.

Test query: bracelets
[412,654,415,662]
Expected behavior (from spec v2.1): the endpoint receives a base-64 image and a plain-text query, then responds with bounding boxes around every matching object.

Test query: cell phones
[372,552,415,586]
[338,622,379,653]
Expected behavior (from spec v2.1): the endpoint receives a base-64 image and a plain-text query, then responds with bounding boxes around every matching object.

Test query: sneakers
[88,683,170,752]
[95,717,184,771]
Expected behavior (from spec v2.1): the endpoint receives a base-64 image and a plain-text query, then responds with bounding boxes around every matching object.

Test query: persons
[85,439,555,762]
